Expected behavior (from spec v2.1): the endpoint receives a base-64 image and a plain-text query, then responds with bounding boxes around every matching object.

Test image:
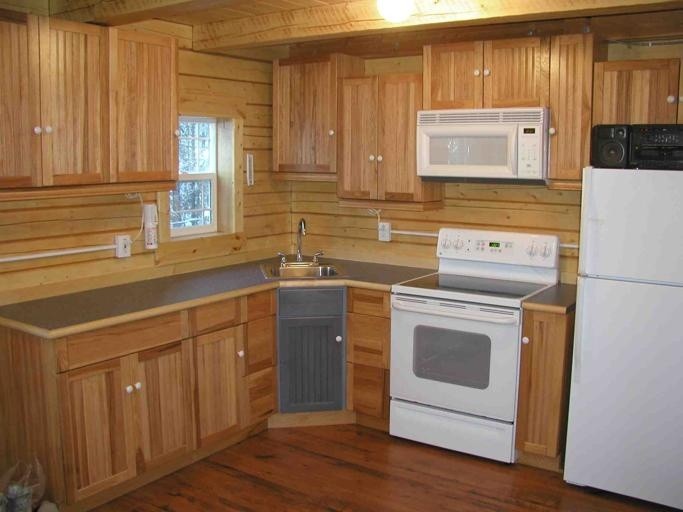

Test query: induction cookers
[399,271,550,300]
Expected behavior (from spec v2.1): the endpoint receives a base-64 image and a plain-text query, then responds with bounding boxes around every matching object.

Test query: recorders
[592,124,683,170]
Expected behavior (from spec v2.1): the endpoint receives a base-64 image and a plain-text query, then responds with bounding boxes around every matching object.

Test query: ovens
[389,293,519,465]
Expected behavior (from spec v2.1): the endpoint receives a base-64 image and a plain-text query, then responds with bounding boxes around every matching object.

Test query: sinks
[266,265,340,279]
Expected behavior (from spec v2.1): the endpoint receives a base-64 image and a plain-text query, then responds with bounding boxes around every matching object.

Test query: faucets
[295,218,307,262]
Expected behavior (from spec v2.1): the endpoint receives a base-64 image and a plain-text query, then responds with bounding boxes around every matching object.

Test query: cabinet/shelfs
[548,33,609,190]
[242,278,279,443]
[336,73,443,211]
[184,288,248,463]
[511,280,579,477]
[106,22,180,199]
[0,304,196,511]
[273,52,366,182]
[0,5,109,198]
[423,36,549,110]
[592,59,682,127]
[339,261,440,435]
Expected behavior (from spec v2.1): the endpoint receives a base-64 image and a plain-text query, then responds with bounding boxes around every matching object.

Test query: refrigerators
[562,165,682,512]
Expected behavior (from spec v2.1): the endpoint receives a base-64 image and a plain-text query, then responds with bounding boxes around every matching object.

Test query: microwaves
[414,107,548,185]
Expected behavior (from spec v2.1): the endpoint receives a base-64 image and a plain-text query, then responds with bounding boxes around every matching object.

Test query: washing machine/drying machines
[389,228,560,466]
